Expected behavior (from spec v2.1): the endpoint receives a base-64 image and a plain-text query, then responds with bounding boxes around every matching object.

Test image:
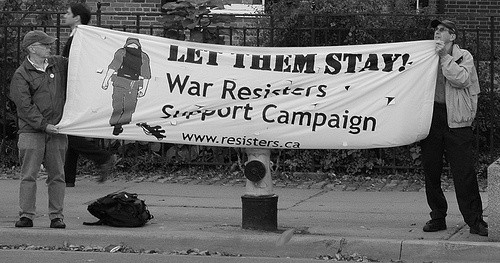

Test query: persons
[60,3,120,186]
[10,30,66,228]
[418,20,490,236]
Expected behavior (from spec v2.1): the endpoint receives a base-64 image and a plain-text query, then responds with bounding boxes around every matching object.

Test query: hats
[22,30,58,51]
[430,19,459,39]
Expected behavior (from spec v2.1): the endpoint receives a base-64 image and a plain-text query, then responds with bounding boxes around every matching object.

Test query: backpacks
[83,191,155,228]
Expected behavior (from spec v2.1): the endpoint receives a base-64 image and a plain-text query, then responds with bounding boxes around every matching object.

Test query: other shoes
[422,217,447,232]
[50,218,66,228]
[469,215,488,236]
[45,178,76,187]
[15,217,33,227]
[96,154,116,183]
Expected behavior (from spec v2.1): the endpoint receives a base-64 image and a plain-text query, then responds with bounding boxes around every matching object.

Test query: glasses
[31,43,54,46]
[433,27,450,32]
